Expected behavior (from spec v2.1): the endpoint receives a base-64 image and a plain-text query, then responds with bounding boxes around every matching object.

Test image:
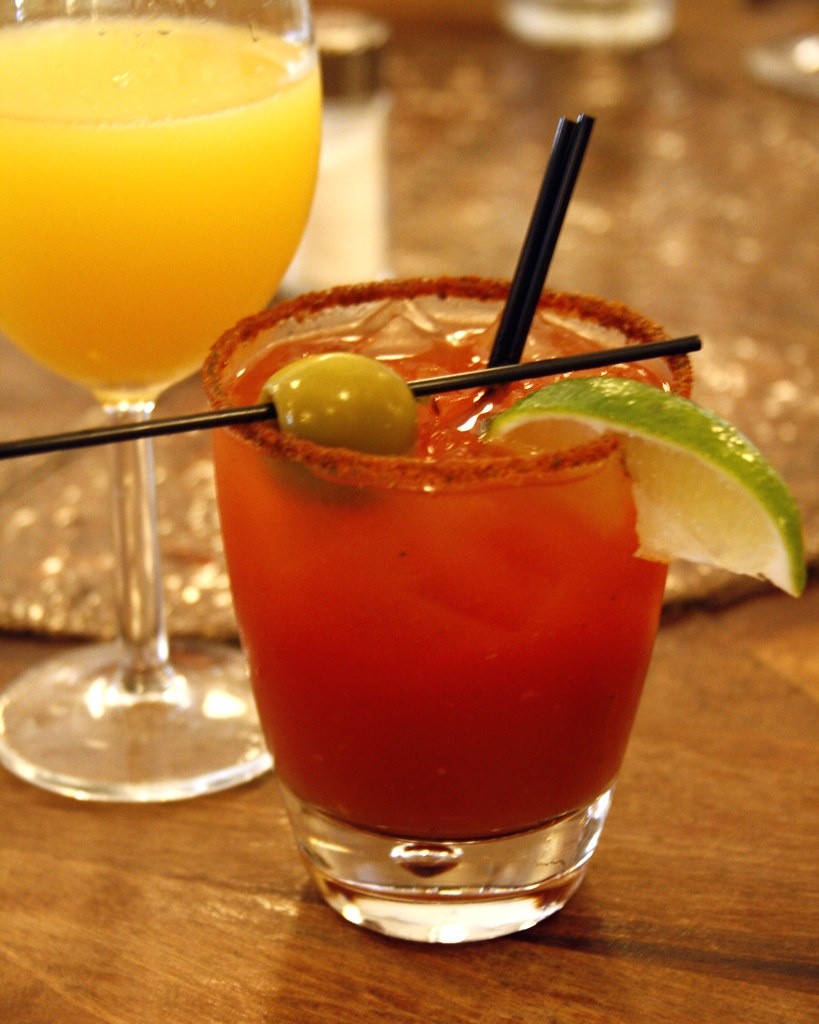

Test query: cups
[200,276,695,945]
[737,0,819,96]
[496,2,675,227]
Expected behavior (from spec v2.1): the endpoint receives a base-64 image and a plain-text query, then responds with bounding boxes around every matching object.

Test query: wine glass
[2,1,322,805]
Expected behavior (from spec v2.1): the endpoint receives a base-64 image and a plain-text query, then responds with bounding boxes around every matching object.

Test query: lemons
[480,378,804,599]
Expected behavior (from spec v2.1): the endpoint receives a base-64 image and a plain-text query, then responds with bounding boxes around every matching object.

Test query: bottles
[279,10,387,290]
